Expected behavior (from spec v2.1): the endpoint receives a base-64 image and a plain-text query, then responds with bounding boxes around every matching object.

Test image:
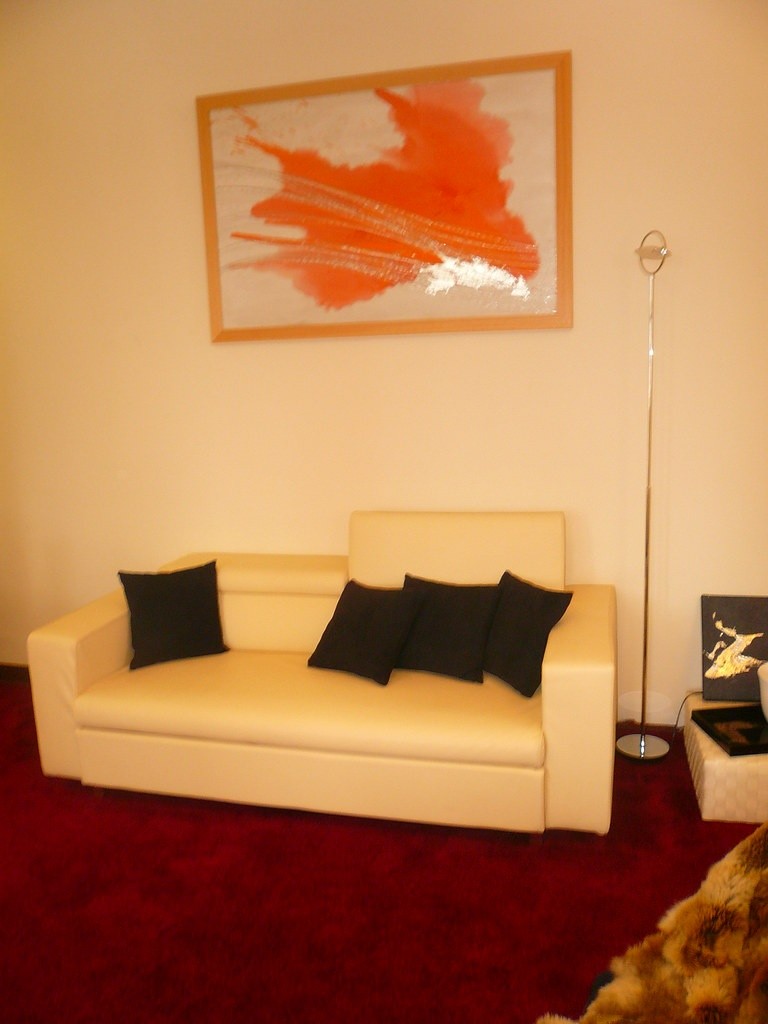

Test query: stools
[682,691,768,823]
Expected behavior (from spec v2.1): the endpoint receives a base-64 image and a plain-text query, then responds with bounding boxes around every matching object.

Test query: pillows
[482,569,574,696]
[308,578,424,685]
[401,572,503,685]
[117,558,230,671]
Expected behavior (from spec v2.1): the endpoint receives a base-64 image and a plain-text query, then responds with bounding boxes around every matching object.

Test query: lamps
[617,231,673,759]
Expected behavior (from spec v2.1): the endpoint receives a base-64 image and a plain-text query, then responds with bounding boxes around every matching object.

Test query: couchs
[28,509,618,835]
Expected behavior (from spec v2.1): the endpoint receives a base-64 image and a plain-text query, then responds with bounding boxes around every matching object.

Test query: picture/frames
[193,47,577,344]
